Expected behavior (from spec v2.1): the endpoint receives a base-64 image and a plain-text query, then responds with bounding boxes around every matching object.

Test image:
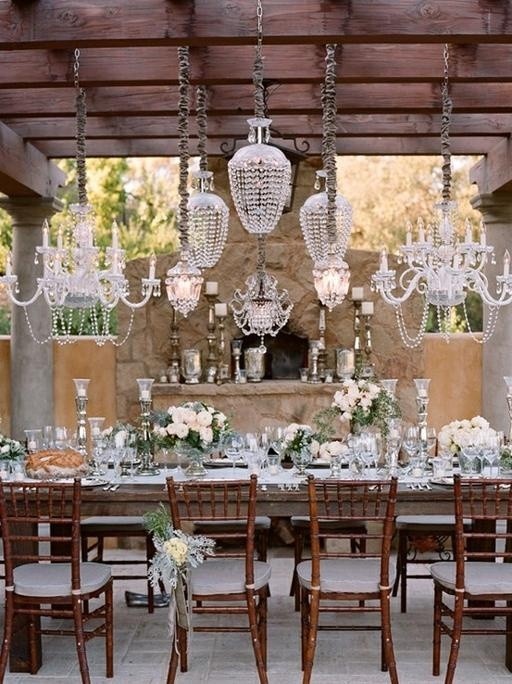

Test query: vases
[454,448,487,474]
[173,442,220,478]
[290,446,313,479]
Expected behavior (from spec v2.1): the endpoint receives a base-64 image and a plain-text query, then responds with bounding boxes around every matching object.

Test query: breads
[25,450,88,472]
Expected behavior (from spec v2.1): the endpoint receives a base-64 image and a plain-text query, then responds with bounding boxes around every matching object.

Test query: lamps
[1,86,161,347]
[165,43,353,337]
[370,100,512,349]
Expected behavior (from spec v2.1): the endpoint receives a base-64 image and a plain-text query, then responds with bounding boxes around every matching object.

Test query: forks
[103,482,512,493]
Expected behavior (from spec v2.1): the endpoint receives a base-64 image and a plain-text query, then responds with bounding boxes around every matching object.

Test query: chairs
[429,473,512,684]
[164,474,272,684]
[297,475,399,684]
[79,515,473,614]
[0,476,115,684]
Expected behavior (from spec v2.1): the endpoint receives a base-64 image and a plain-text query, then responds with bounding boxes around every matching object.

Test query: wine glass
[1,412,509,481]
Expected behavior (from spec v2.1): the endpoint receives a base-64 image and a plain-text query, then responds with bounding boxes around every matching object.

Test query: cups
[206,368,216,385]
[324,369,335,384]
[300,368,311,383]
[158,367,168,383]
[238,370,247,385]
[336,348,355,382]
[180,348,202,384]
[169,367,177,384]
[244,349,265,383]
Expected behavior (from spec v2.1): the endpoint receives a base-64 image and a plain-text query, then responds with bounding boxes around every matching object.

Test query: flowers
[280,425,324,456]
[150,400,233,452]
[439,416,499,457]
[313,374,404,426]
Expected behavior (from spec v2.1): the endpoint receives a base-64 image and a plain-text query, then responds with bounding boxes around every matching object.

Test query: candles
[420,389,427,397]
[141,390,149,398]
[78,388,86,396]
[353,287,373,316]
